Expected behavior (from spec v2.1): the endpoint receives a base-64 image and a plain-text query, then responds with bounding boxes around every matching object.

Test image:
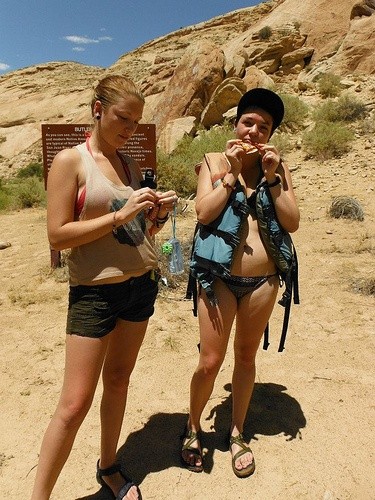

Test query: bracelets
[113,210,118,234]
[221,177,235,191]
[154,212,170,224]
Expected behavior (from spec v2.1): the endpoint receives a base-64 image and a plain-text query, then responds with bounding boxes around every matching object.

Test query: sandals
[96,458,135,500]
[181,424,204,472]
[228,432,256,477]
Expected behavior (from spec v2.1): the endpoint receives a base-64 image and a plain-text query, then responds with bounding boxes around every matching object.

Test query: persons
[181,87,300,478]
[30,76,180,500]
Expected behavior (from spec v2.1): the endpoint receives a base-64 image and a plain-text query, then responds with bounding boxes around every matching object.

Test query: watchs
[262,174,283,189]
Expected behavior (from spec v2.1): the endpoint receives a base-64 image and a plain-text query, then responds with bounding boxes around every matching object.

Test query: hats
[233,88,285,143]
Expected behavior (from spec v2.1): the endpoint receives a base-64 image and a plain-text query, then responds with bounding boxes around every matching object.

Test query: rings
[269,154,274,159]
